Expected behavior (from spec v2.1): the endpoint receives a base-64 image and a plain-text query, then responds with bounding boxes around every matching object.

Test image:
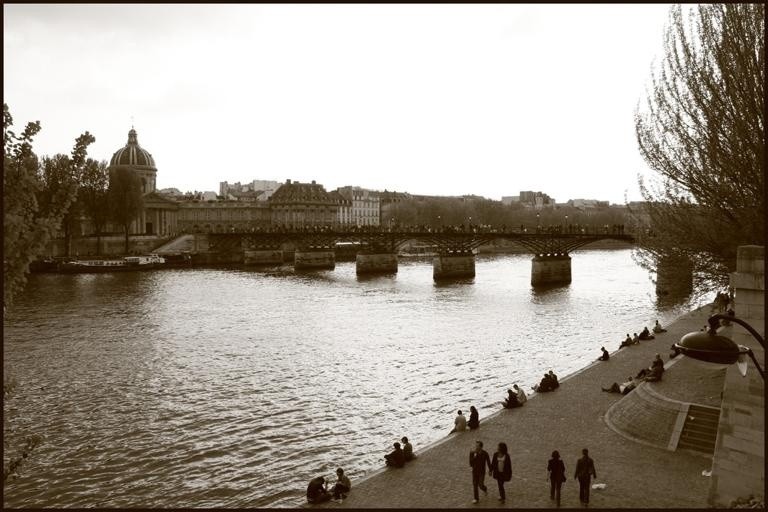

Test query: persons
[467,406,478,429]
[598,347,609,360]
[622,320,661,346]
[547,450,566,505]
[469,441,491,504]
[385,437,413,468]
[502,384,526,408]
[330,468,351,504]
[670,344,680,359]
[532,371,559,393]
[602,376,635,395]
[636,353,664,380]
[574,449,596,505]
[491,442,512,504]
[307,475,331,503]
[717,292,728,312]
[450,410,466,434]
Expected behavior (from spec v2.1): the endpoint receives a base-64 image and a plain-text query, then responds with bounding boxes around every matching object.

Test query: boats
[48,253,165,274]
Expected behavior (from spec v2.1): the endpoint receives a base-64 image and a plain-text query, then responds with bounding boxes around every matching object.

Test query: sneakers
[497,498,506,504]
[484,491,487,496]
[472,499,479,503]
